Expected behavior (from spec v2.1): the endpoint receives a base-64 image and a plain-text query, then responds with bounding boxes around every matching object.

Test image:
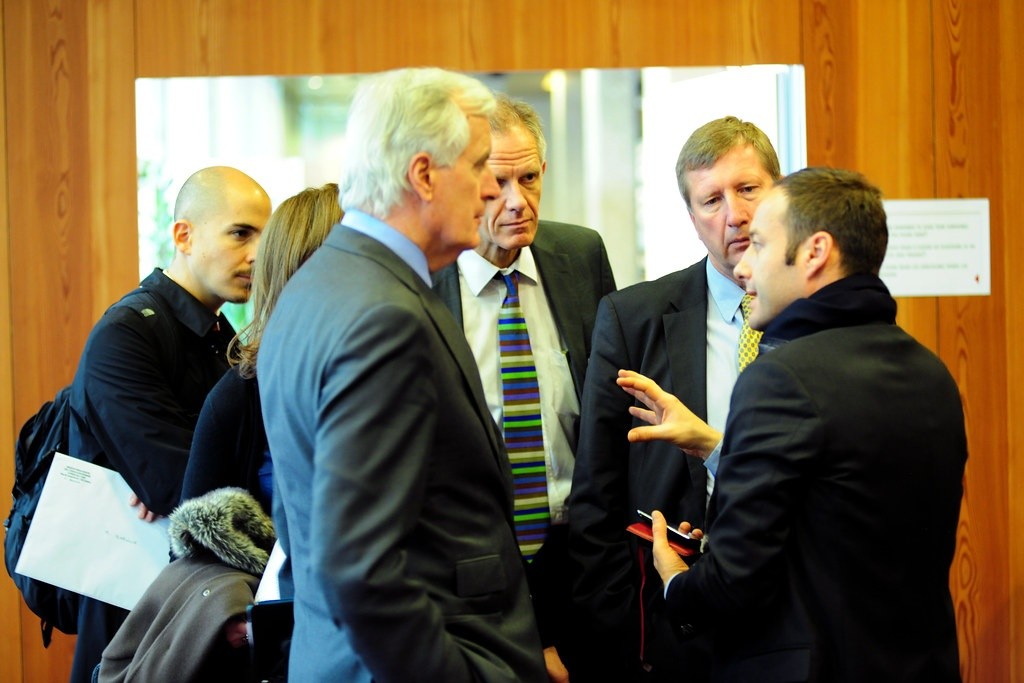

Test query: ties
[739,293,764,376]
[488,270,553,566]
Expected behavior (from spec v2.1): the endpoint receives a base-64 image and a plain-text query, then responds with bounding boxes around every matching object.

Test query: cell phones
[636,509,701,549]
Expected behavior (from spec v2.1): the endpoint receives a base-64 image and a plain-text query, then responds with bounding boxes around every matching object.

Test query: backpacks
[4,294,190,649]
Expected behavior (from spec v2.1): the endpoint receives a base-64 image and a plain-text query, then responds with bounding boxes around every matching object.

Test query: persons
[530,117,786,683]
[167,185,345,683]
[71,166,273,682]
[617,167,970,683]
[458,95,618,570]
[257,68,551,683]
